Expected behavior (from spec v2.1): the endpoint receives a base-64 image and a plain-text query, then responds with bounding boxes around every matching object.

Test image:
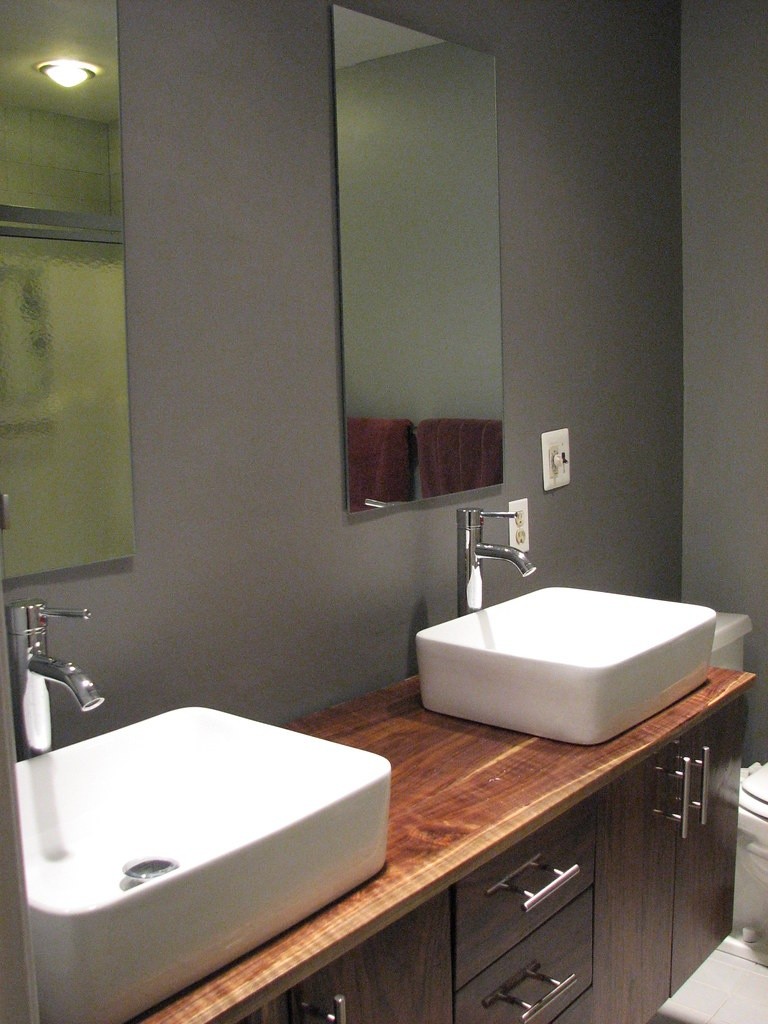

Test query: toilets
[710,611,767,944]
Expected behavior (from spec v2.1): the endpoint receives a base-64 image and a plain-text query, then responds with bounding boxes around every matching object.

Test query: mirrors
[0,0,137,580]
[326,2,505,528]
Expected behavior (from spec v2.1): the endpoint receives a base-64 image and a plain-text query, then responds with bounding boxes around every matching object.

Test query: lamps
[32,59,101,89]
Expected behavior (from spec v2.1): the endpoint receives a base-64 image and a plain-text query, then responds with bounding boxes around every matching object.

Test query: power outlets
[507,497,532,552]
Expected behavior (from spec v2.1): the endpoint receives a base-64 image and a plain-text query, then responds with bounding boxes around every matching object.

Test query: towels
[346,416,417,509]
[415,417,505,500]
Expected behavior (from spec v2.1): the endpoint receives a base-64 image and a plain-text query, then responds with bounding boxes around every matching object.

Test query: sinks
[16,706,392,1024]
[415,586,717,746]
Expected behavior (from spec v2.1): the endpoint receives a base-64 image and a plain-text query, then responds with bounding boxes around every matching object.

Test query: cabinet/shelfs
[140,666,758,1024]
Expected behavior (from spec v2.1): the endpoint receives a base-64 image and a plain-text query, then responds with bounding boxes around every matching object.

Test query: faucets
[4,597,108,763]
[455,507,538,620]
